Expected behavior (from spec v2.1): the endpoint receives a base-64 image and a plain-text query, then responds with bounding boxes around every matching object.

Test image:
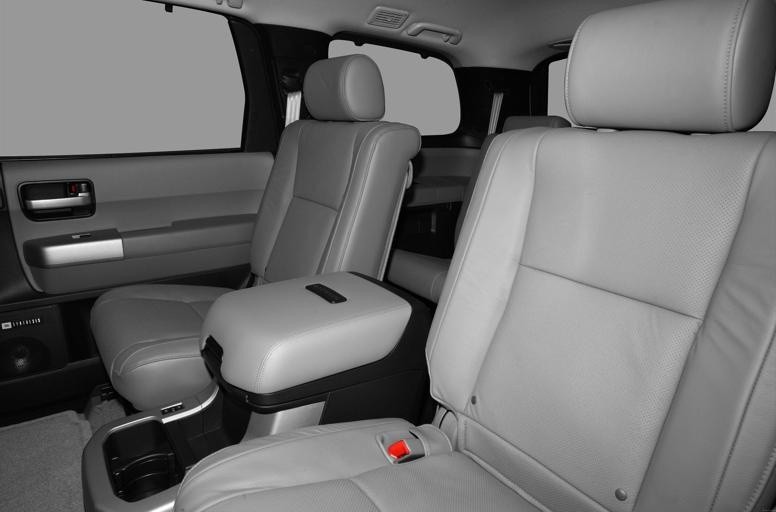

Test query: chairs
[88,54,426,416]
[383,114,574,309]
[173,2,774,512]
